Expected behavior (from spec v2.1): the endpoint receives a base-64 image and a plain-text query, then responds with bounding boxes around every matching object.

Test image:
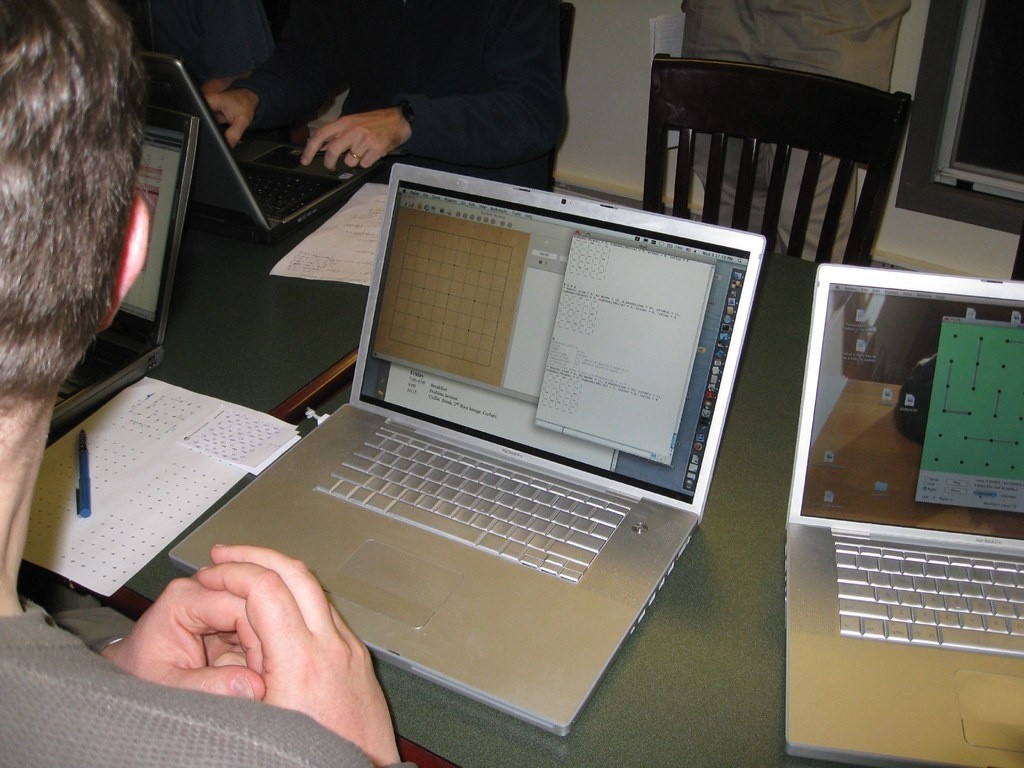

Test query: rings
[349,150,361,160]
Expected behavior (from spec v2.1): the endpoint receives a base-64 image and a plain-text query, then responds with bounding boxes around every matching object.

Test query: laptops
[168,162,768,735]
[785,263,1024,768]
[50,107,198,424]
[134,52,370,233]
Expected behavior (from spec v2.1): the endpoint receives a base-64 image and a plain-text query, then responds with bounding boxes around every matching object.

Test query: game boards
[372,206,531,386]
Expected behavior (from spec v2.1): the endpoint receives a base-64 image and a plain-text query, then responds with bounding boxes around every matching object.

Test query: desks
[15,176,909,768]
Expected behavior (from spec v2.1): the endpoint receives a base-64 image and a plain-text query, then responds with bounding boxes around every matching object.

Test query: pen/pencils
[75,425,93,518]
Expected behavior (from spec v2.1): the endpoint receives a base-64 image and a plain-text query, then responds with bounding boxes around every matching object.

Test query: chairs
[642,53,912,267]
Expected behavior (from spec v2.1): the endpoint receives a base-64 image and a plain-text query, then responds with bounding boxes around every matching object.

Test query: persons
[682,0,915,267]
[194,0,574,196]
[115,1,275,101]
[0,0,413,768]
